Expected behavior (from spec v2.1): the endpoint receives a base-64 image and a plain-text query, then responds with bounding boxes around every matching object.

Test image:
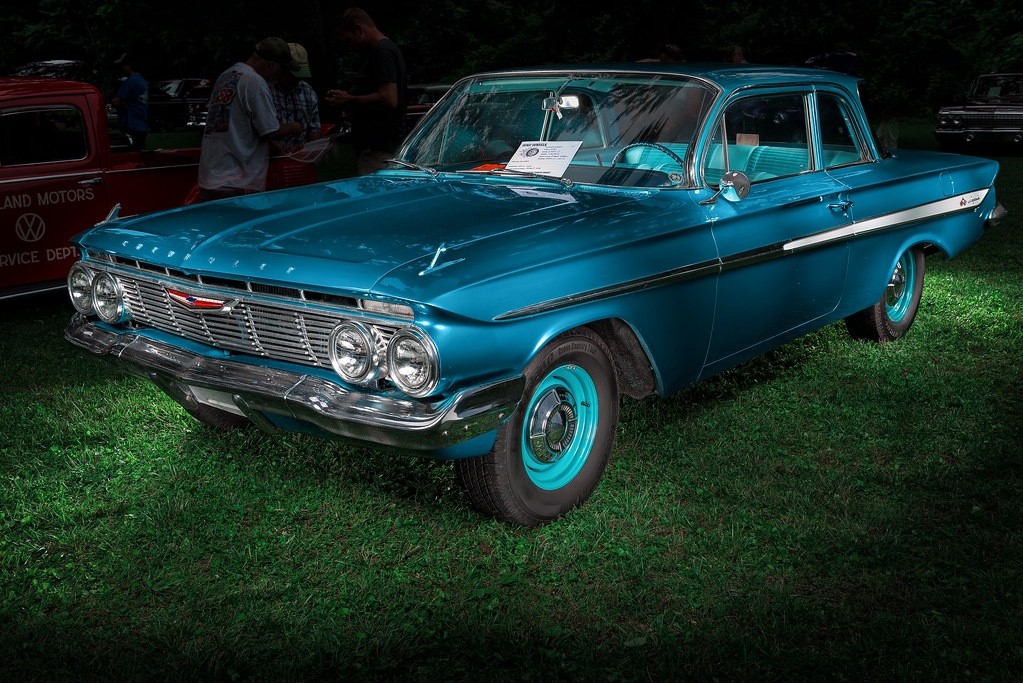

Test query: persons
[269,41,320,154]
[197,36,301,202]
[730,46,748,65]
[111,52,150,149]
[326,8,410,172]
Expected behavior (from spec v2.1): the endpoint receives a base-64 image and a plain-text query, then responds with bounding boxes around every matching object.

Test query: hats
[113,53,136,65]
[256,36,301,71]
[287,42,312,78]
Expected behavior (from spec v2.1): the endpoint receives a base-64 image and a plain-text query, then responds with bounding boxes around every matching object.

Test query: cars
[935,69,1023,157]
[61,66,1009,531]
[13,59,453,140]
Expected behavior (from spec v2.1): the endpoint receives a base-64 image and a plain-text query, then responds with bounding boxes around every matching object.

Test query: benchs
[625,143,863,177]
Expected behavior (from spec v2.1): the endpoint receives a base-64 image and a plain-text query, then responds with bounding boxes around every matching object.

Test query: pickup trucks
[0,76,336,301]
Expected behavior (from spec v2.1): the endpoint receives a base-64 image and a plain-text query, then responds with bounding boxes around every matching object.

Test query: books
[287,133,334,163]
